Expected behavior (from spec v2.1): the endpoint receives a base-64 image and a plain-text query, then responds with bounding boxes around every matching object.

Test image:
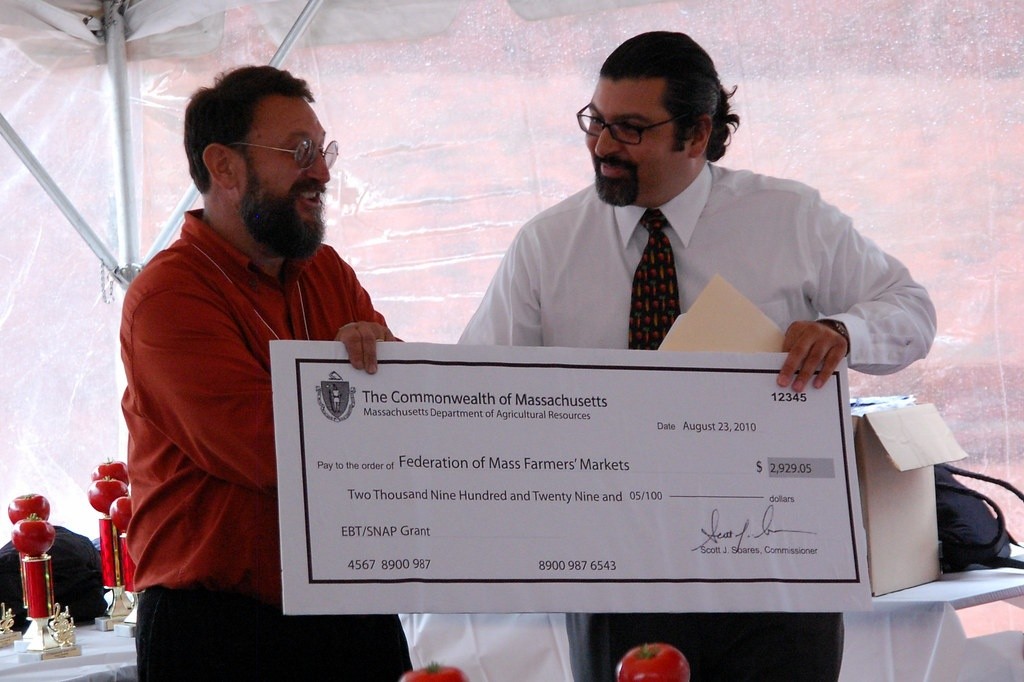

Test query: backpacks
[932,461,1024,573]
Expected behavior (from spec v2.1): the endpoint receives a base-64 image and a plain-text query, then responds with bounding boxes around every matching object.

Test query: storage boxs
[851,404,969,597]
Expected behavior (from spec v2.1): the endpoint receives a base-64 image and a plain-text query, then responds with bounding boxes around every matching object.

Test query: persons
[457,31,937,682]
[122,65,414,682]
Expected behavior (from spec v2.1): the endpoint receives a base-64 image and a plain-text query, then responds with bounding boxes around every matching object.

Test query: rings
[376,339,384,342]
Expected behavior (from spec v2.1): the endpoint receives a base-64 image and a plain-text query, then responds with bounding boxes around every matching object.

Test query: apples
[399,661,469,682]
[616,643,690,682]
[87,458,133,533]
[7,494,55,557]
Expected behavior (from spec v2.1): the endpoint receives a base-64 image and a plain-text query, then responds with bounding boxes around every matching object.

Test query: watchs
[830,320,848,336]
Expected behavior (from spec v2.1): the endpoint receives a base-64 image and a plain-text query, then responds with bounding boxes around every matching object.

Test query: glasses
[223,138,339,170]
[576,102,686,145]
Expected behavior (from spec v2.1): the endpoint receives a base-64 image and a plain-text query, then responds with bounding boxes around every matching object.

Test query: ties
[628,209,682,350]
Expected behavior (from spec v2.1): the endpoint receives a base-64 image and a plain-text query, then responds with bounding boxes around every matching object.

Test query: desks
[398,540,1024,682]
[0,621,138,682]
